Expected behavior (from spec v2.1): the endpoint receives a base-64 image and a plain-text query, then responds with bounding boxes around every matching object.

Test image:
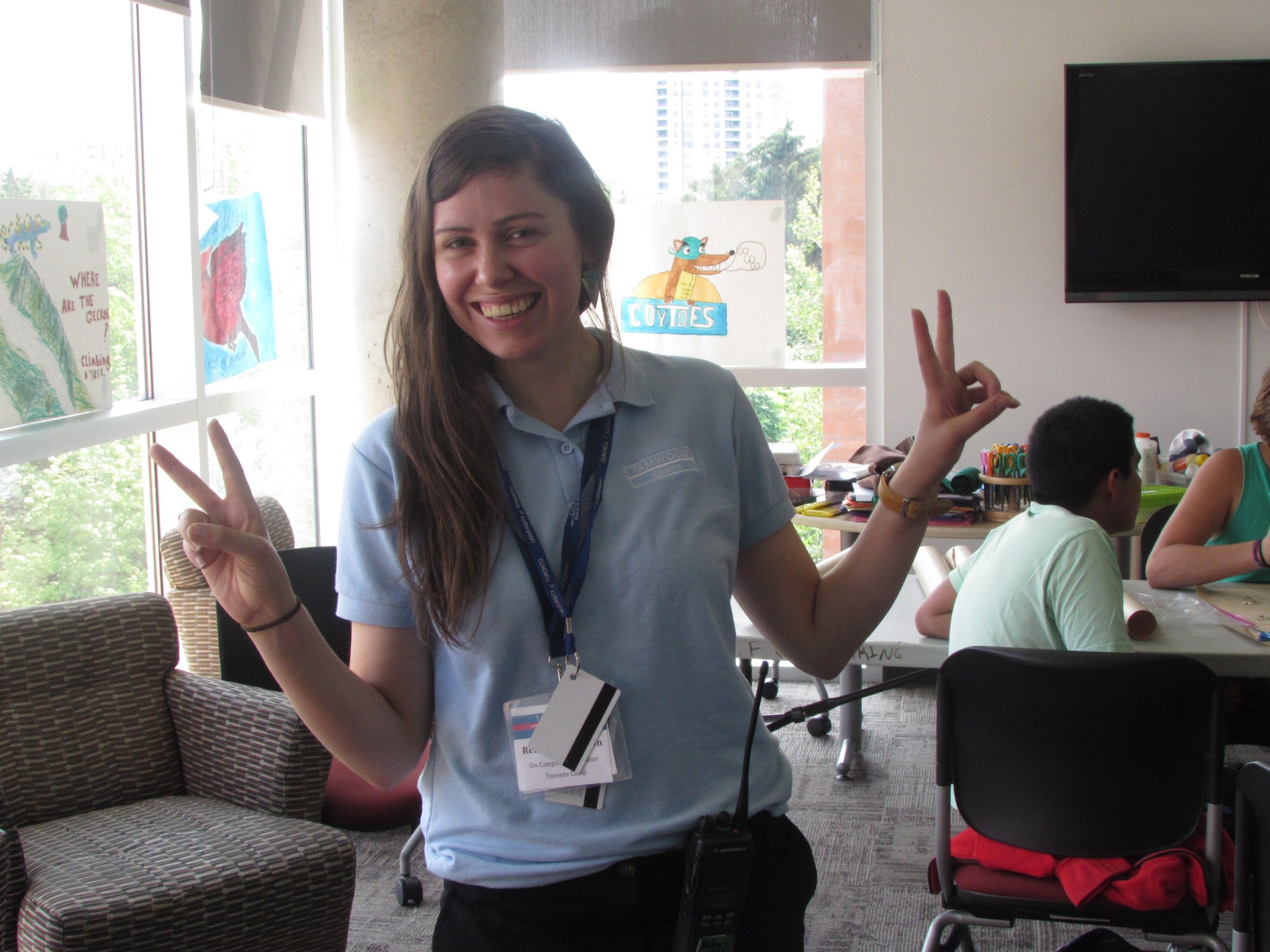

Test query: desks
[919,503,1270,952]
[730,569,1270,676]
[789,507,1145,781]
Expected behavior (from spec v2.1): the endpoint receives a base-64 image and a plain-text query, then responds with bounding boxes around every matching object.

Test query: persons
[147,107,1020,952]
[1145,366,1270,591]
[916,398,1142,809]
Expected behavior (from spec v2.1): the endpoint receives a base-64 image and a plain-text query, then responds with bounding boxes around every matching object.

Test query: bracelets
[240,594,301,632]
[1253,538,1270,568]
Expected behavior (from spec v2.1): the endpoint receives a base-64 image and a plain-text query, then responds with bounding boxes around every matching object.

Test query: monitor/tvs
[1064,59,1270,304]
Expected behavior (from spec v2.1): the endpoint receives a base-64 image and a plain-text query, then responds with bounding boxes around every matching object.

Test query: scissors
[981,443,1031,510]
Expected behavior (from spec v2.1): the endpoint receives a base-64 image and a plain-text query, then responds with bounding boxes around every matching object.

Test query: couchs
[1,494,431,952]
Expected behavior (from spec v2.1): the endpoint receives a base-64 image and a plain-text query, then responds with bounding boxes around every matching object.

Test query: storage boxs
[1137,484,1188,525]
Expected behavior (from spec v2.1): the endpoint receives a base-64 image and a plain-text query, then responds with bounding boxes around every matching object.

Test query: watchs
[876,462,953,519]
